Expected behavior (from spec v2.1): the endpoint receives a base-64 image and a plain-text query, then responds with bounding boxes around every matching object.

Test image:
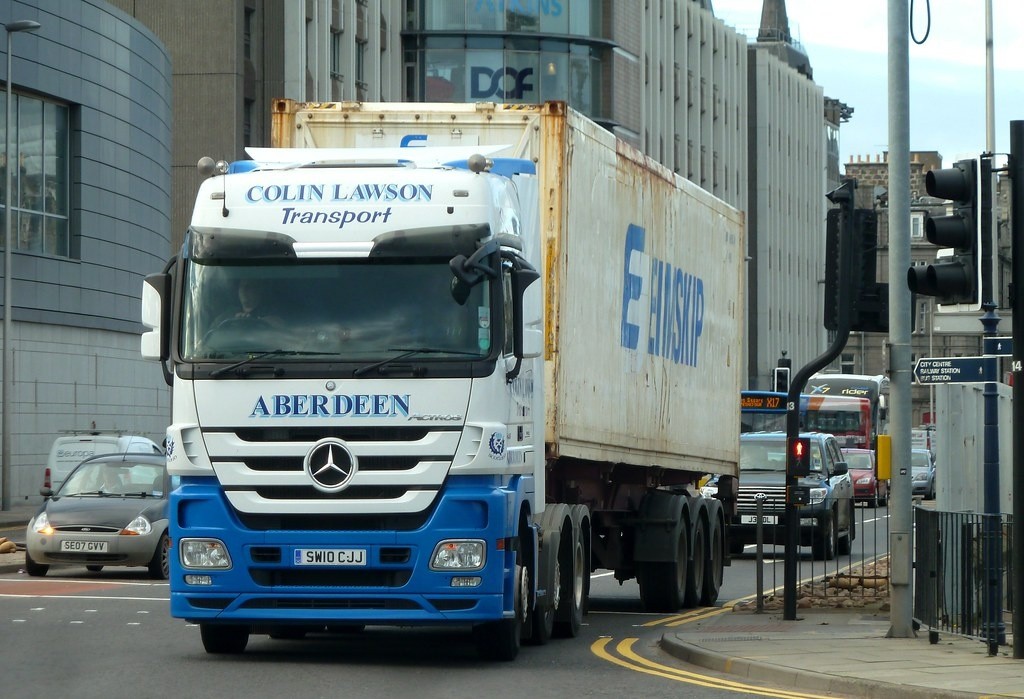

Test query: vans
[42,433,164,501]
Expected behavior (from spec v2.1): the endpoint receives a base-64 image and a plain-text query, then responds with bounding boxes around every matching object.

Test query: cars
[839,448,887,507]
[909,450,937,499]
[24,452,169,578]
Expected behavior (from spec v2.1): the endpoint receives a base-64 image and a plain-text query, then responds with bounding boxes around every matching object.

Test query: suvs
[699,429,854,560]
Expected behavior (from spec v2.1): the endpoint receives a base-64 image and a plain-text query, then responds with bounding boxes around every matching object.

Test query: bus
[804,370,892,448]
[741,391,871,449]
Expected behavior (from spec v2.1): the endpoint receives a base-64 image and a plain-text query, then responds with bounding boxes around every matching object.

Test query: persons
[209,276,280,327]
[97,468,123,494]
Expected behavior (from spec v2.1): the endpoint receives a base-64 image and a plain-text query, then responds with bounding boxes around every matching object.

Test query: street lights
[2,20,39,510]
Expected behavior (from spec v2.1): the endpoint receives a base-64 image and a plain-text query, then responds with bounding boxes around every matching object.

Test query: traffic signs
[912,355,998,387]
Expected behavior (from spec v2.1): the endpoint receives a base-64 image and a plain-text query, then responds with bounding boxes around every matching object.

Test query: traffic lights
[904,157,992,313]
[784,435,810,476]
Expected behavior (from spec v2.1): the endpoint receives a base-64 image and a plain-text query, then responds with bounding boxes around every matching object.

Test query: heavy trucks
[140,101,749,659]
